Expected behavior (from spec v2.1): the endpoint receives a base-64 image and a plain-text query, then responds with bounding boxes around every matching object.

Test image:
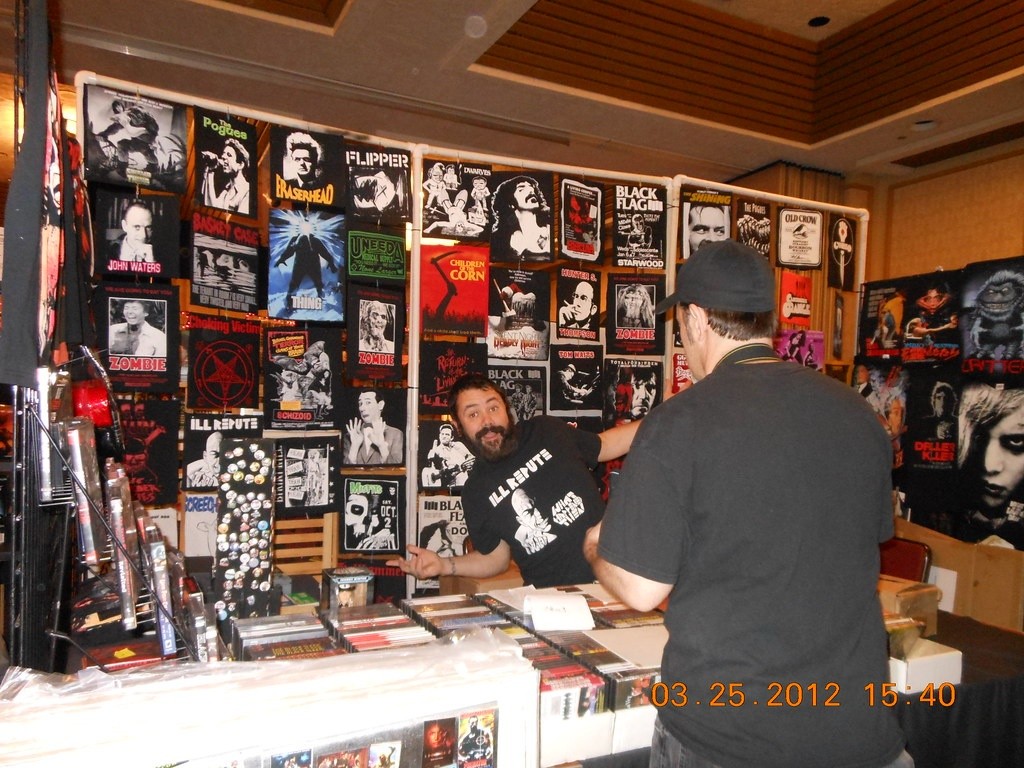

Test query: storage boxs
[878,573,937,637]
[888,638,962,695]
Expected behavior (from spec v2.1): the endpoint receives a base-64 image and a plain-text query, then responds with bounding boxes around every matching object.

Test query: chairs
[879,536,932,583]
[273,512,338,615]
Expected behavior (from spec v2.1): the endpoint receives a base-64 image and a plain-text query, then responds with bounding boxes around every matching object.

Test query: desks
[891,612,1024,768]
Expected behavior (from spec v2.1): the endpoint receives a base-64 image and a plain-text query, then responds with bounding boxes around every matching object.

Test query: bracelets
[449,556,456,576]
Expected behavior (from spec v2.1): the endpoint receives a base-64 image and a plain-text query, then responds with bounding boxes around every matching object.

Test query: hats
[653,237,774,315]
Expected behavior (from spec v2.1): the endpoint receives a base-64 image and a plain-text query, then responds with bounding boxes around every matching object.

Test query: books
[231,582,665,768]
[883,613,926,660]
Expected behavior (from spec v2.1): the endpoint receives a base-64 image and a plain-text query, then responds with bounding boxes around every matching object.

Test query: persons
[781,330,806,364]
[109,300,166,357]
[459,715,493,761]
[342,387,403,465]
[586,239,914,768]
[202,139,250,215]
[427,424,474,487]
[558,280,597,330]
[429,724,446,748]
[689,203,725,255]
[958,382,1024,552]
[187,432,223,486]
[286,132,321,189]
[107,202,160,262]
[852,364,872,397]
[385,373,641,589]
